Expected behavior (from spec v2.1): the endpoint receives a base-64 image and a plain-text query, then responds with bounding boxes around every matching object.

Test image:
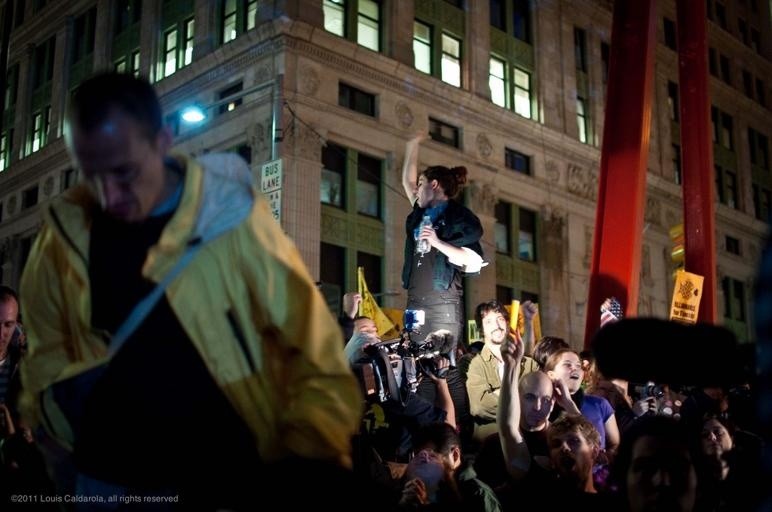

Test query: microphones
[424,329,454,353]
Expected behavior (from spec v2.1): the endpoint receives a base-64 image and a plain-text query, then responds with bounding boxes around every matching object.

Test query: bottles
[416,216,433,253]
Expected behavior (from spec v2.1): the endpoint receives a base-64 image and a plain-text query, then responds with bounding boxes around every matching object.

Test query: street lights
[179,73,281,162]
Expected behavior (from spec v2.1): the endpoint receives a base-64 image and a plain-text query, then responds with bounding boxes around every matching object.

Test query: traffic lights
[669,224,685,283]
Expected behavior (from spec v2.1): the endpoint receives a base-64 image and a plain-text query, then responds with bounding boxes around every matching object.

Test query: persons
[0,70,772,511]
